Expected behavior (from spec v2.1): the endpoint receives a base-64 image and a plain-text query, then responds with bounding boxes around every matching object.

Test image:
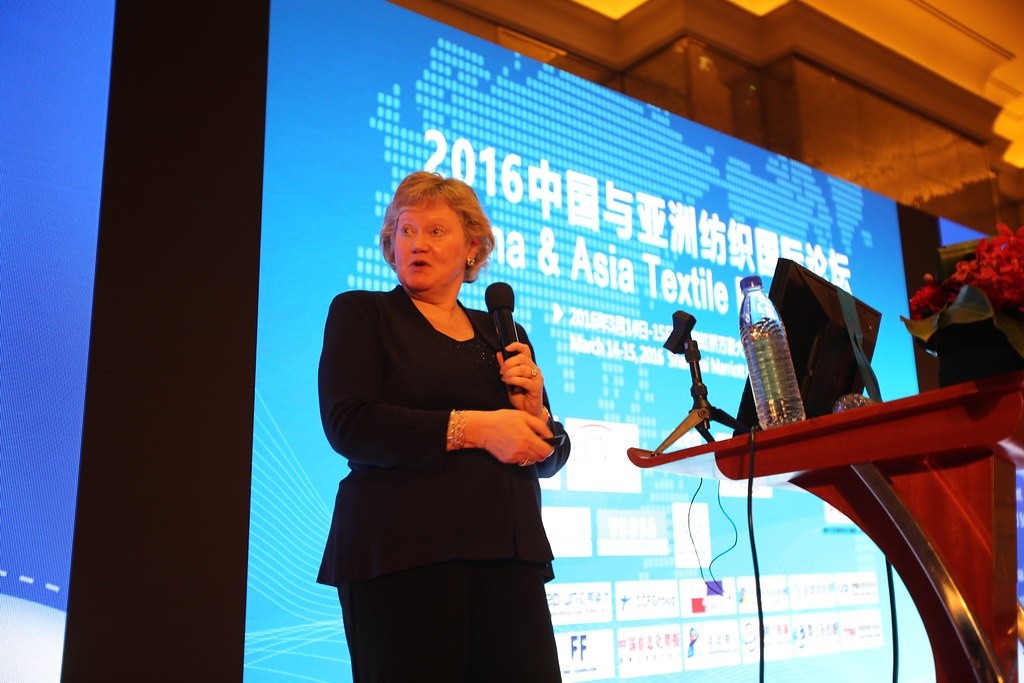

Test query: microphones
[485,282,524,395]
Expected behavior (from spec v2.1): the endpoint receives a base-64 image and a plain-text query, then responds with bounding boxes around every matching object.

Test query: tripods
[650,309,737,458]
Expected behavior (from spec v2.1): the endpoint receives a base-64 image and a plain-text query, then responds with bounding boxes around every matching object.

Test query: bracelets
[543,405,551,427]
[448,408,465,449]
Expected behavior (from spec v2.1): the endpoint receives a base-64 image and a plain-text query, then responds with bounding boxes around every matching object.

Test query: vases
[914,324,1024,387]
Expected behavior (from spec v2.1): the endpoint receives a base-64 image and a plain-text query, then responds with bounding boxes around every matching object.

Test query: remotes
[542,435,566,446]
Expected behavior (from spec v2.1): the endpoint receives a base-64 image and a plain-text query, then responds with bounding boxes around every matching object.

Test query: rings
[521,459,531,466]
[528,368,536,378]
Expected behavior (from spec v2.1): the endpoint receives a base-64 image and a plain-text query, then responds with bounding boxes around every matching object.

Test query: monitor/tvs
[937,238,1024,387]
[732,258,882,438]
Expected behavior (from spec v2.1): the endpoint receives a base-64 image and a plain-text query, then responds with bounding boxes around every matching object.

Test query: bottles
[738,277,806,430]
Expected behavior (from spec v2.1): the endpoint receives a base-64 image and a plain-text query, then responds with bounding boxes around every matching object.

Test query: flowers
[904,225,1024,335]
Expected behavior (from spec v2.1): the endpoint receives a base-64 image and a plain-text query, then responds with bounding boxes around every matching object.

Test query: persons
[315,171,571,683]
